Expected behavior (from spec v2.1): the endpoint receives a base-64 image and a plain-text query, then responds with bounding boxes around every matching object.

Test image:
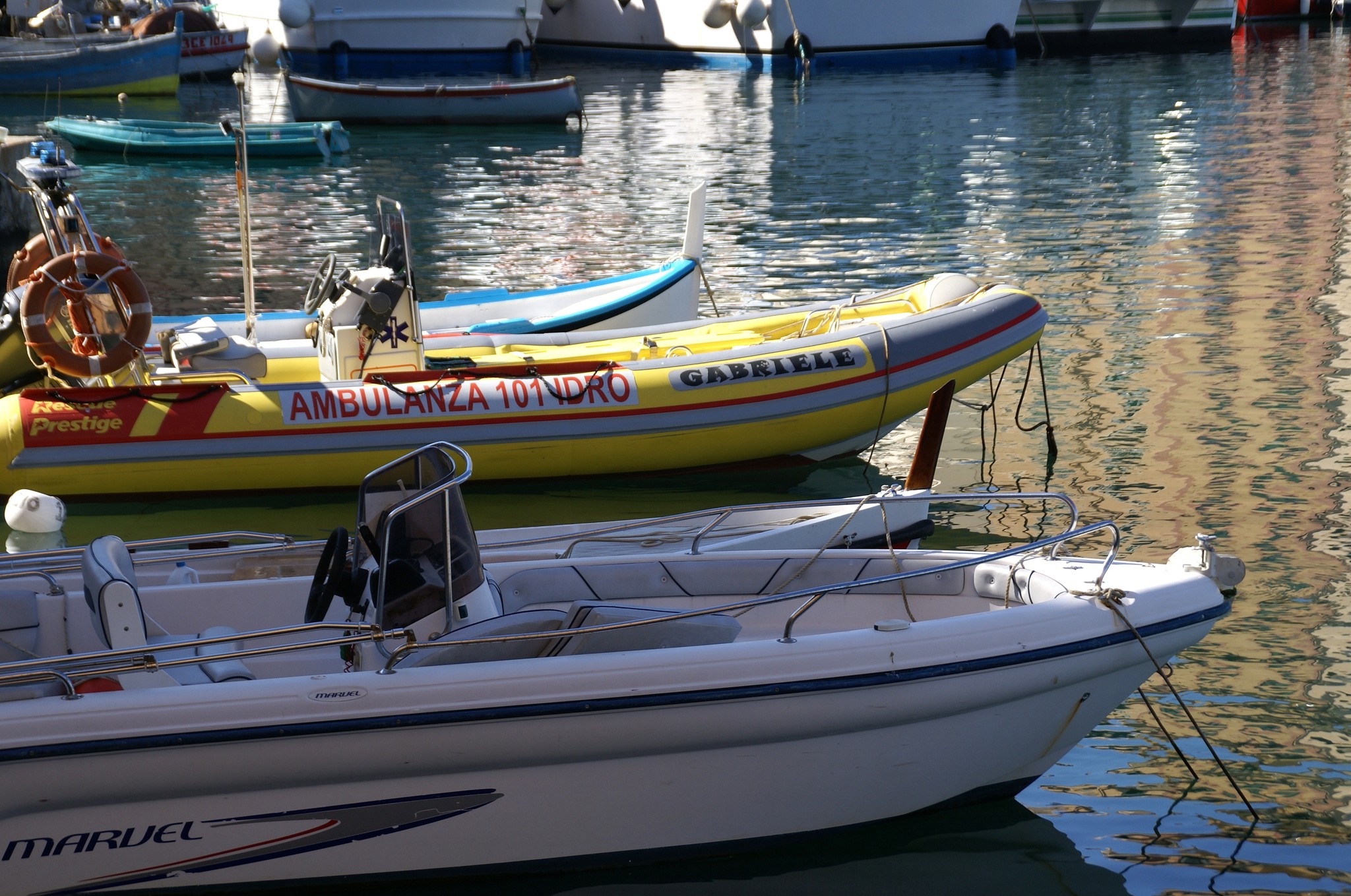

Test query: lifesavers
[55,15,67,31]
[19,249,152,380]
[6,230,129,310]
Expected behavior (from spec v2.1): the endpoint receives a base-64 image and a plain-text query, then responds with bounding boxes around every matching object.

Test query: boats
[0,0,549,76]
[0,533,1253,896]
[0,9,187,96]
[282,68,591,127]
[35,111,350,160]
[536,0,1024,81]
[115,183,719,358]
[41,24,253,82]
[1,138,1052,510]
[2,372,963,609]
